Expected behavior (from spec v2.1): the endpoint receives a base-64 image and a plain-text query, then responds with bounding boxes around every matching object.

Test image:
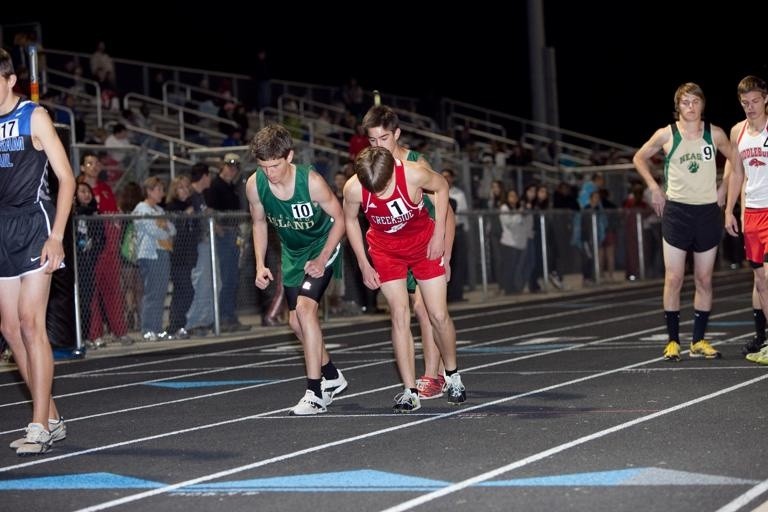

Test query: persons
[341,143,468,410]
[0,47,78,457]
[46,121,288,351]
[358,102,458,402]
[184,51,304,148]
[314,86,381,315]
[5,33,121,144]
[547,150,666,290]
[722,196,746,271]
[407,111,550,304]
[717,75,767,363]
[631,81,745,361]
[243,123,351,417]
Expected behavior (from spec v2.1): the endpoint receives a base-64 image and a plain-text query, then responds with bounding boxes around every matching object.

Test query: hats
[223,153,241,165]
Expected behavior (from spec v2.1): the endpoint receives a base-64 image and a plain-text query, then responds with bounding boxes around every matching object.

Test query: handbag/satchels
[122,225,139,265]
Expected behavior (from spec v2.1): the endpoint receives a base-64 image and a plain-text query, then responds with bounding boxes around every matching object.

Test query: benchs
[54,91,431,196]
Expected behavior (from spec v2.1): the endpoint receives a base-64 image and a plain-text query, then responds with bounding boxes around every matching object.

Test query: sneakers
[664,342,681,360]
[290,391,328,414]
[231,321,252,331]
[688,342,720,359]
[81,334,134,350]
[10,418,66,448]
[18,431,52,455]
[393,388,420,411]
[262,316,278,327]
[144,328,204,341]
[418,373,468,405]
[743,338,768,364]
[211,324,237,333]
[319,370,349,405]
[273,316,286,326]
